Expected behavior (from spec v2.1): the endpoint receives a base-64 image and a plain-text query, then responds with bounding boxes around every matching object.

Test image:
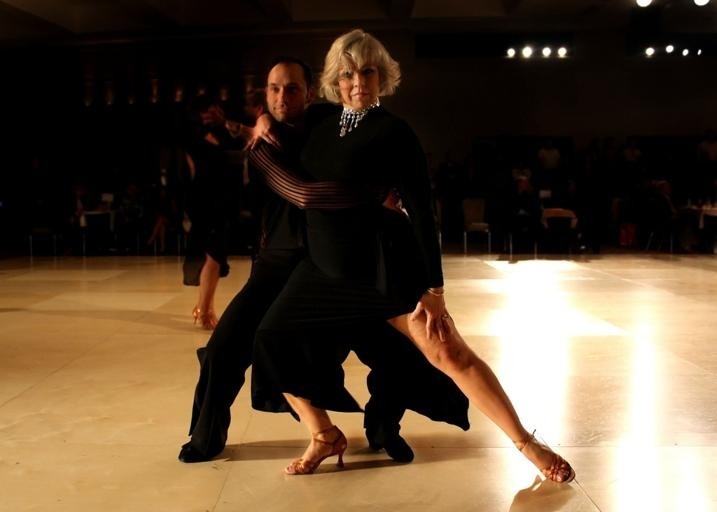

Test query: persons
[243,27,578,486]
[177,55,472,464]
[61,74,269,333]
[421,126,717,254]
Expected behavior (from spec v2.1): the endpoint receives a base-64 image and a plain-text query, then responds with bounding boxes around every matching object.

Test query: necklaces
[336,101,381,136]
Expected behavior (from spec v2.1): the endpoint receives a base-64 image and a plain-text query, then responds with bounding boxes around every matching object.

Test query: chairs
[461,195,492,253]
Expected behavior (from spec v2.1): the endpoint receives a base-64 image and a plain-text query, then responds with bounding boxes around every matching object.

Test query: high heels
[193,305,219,330]
[366,429,414,463]
[179,440,225,462]
[285,426,347,475]
[515,430,575,483]
